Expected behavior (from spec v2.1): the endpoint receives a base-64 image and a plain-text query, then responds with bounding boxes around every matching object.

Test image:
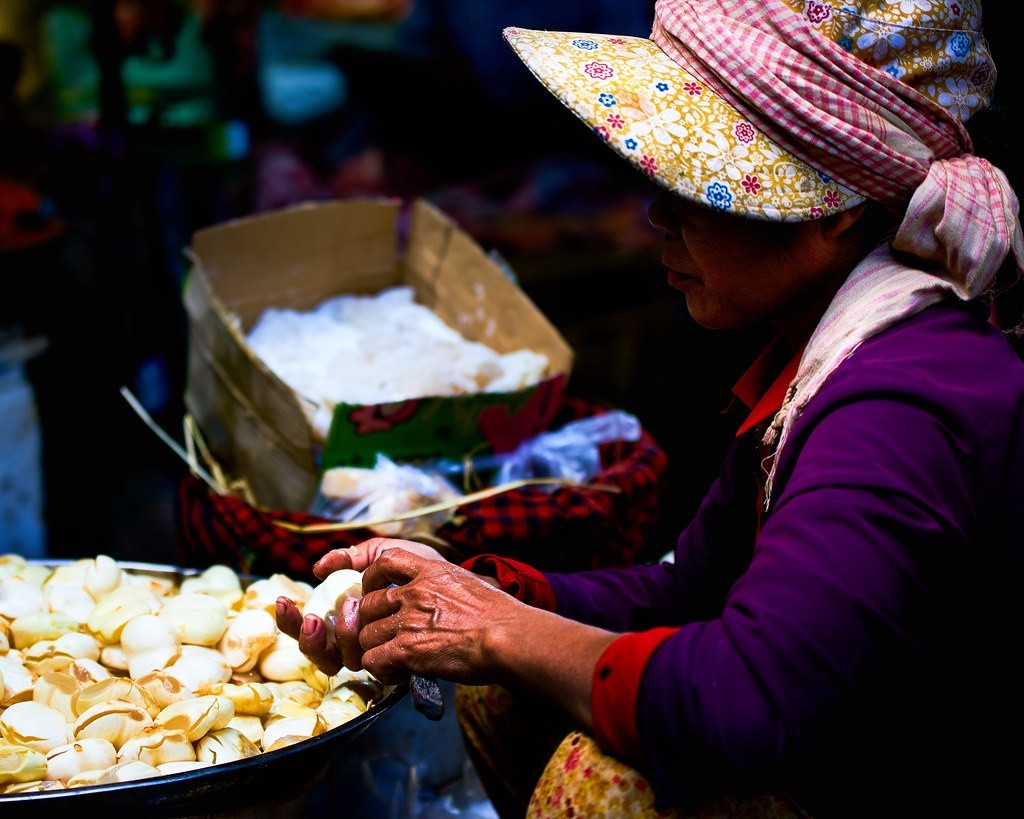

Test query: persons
[260,0,1024,819]
[0,33,69,562]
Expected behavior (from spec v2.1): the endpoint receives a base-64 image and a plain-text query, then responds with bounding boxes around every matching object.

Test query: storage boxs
[174,197,575,521]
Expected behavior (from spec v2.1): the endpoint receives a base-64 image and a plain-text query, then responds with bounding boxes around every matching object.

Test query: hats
[502,0,998,223]
